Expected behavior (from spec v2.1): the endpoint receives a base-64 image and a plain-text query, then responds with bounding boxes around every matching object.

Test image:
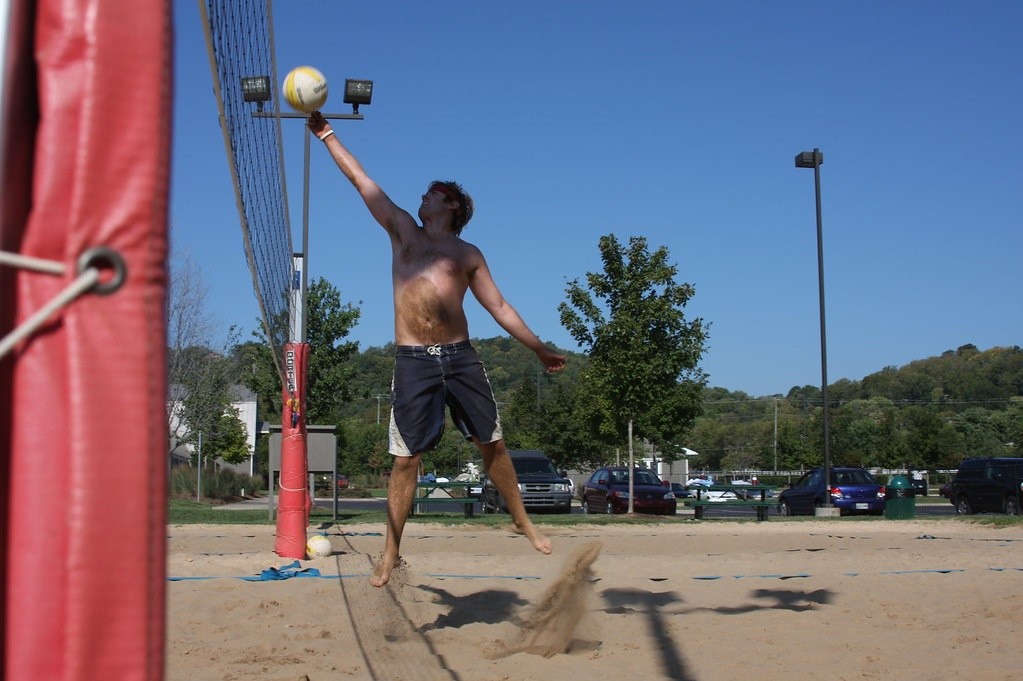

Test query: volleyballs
[282,65,328,114]
[306,535,332,561]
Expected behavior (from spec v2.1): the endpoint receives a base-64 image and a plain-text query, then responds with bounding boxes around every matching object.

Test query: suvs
[949,457,1023,516]
[482,449,572,514]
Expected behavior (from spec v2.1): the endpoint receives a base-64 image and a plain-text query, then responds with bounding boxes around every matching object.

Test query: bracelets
[319,130,334,141]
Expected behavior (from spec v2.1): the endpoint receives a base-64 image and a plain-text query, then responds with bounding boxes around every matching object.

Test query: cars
[939,479,953,499]
[911,477,930,495]
[669,481,691,499]
[581,467,676,516]
[777,466,886,518]
[690,477,774,506]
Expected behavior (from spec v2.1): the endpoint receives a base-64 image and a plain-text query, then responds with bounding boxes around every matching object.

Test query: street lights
[795,147,841,518]
[239,72,375,352]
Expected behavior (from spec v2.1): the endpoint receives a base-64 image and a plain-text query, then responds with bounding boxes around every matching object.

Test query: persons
[308,111,566,587]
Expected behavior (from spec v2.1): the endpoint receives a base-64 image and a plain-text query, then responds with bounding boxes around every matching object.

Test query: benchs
[683,483,782,520]
[411,481,481,517]
